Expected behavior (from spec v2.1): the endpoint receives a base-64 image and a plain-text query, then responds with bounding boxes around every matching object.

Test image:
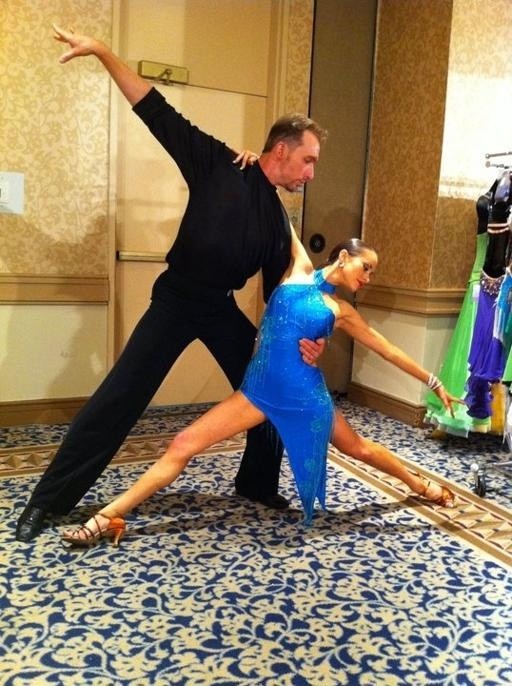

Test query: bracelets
[427,372,443,391]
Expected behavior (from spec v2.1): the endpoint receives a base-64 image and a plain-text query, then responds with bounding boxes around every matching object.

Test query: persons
[12,23,330,543]
[61,149,469,549]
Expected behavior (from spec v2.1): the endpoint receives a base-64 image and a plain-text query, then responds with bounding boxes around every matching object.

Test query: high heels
[64,512,126,547]
[410,472,454,507]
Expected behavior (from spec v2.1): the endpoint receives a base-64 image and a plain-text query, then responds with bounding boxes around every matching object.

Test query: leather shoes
[17,499,48,541]
[236,479,289,508]
